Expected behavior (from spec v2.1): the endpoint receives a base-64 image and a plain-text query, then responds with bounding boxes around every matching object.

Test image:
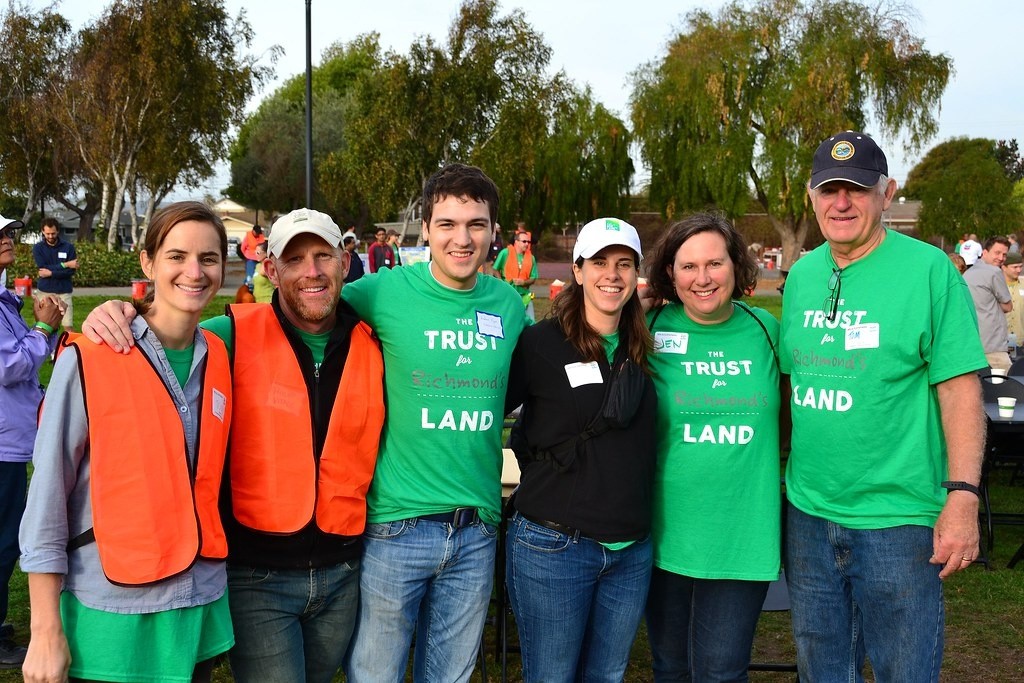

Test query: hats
[810,130,888,188]
[266,208,345,260]
[1004,252,1022,266]
[573,217,643,271]
[0,214,25,230]
[387,229,400,239]
[495,223,500,229]
[253,225,262,234]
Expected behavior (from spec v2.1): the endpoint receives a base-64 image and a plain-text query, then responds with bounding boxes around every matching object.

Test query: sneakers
[0,624,17,637]
[0,639,28,670]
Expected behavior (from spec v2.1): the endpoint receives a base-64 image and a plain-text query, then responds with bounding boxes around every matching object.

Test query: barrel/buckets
[637,284,650,294]
[132,280,148,300]
[14,278,32,296]
[550,283,563,300]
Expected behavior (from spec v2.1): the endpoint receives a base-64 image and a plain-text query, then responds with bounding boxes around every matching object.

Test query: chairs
[942,355,1024,571]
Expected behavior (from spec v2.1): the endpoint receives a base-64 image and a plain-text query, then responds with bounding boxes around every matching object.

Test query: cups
[991,369,1005,384]
[998,397,1017,417]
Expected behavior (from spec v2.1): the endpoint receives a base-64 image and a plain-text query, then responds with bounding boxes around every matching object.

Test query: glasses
[0,227,16,240]
[823,267,842,321]
[520,240,530,243]
[377,234,385,236]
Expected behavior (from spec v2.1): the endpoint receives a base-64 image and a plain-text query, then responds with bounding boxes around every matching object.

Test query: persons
[240,224,276,303]
[643,213,791,683]
[341,162,664,683]
[780,130,989,683]
[0,214,68,670]
[81,208,386,683]
[32,218,81,361]
[504,217,659,683]
[342,223,402,286]
[22,202,236,683]
[947,233,1024,372]
[486,223,539,326]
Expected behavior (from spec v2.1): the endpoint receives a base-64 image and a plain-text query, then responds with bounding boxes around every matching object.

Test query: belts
[518,512,647,543]
[417,508,479,529]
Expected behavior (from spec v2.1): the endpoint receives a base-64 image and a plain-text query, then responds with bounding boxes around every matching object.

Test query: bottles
[1007,332,1017,358]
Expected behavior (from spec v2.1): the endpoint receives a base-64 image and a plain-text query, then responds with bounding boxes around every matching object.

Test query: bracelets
[523,280,526,285]
[941,482,979,497]
[34,322,54,334]
[61,262,66,268]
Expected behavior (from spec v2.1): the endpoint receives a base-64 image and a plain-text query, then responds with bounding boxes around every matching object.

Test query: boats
[754,246,814,272]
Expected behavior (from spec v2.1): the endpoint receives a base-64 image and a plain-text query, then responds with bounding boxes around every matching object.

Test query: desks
[762,252,783,268]
[980,402,1024,525]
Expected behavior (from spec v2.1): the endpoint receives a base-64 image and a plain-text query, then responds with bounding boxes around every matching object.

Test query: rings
[962,557,972,561]
